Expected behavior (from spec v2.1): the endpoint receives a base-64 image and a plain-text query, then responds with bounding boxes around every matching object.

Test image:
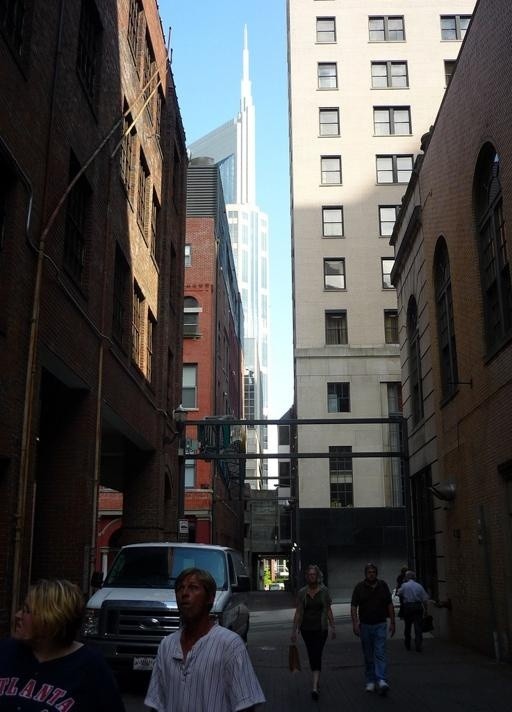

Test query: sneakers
[311,687,322,697]
[365,676,391,693]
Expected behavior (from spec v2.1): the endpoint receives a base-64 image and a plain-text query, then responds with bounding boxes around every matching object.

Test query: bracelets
[391,620,395,624]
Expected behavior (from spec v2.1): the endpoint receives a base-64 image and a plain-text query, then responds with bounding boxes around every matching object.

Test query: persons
[287,564,337,696]
[351,562,395,691]
[143,567,268,710]
[397,570,434,652]
[397,567,408,619]
[0,578,123,711]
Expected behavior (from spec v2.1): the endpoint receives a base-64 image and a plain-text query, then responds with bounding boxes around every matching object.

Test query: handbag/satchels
[288,642,302,677]
[420,613,434,633]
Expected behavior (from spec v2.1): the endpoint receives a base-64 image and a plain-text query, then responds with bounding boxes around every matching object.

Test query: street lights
[174,402,188,543]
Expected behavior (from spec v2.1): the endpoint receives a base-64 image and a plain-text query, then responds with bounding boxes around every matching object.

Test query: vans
[84,538,252,681]
